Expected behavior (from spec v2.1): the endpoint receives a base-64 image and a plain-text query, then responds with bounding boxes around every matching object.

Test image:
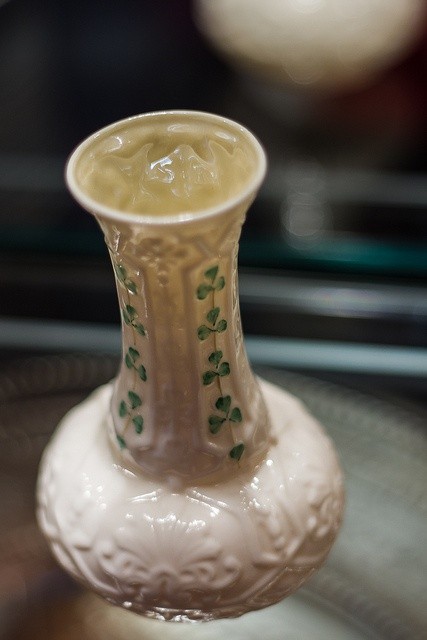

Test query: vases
[37,110,349,623]
